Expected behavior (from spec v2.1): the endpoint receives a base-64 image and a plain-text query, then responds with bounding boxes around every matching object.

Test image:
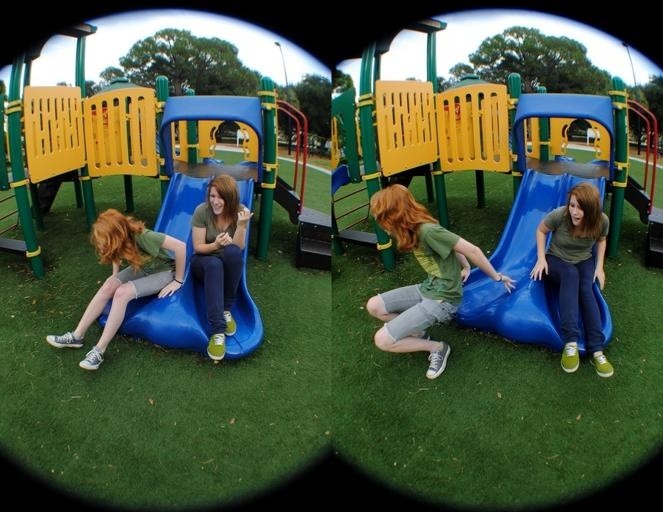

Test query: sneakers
[207,333,226,361]
[560,342,579,373]
[589,352,614,378]
[426,340,450,380]
[78,345,105,371]
[46,331,85,348]
[418,330,430,340]
[224,310,237,337]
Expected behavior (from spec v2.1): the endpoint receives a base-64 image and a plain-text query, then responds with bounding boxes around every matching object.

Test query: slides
[97,172,264,359]
[455,167,613,354]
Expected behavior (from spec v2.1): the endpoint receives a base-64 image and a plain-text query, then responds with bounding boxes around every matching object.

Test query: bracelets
[497,276,503,282]
[173,278,184,286]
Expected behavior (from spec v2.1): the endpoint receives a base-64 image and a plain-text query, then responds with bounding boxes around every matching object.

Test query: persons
[528,182,615,378]
[190,174,255,362]
[364,184,517,379]
[44,208,187,372]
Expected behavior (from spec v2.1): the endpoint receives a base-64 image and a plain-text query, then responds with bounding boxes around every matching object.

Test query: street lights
[621,43,641,155]
[275,41,292,156]
